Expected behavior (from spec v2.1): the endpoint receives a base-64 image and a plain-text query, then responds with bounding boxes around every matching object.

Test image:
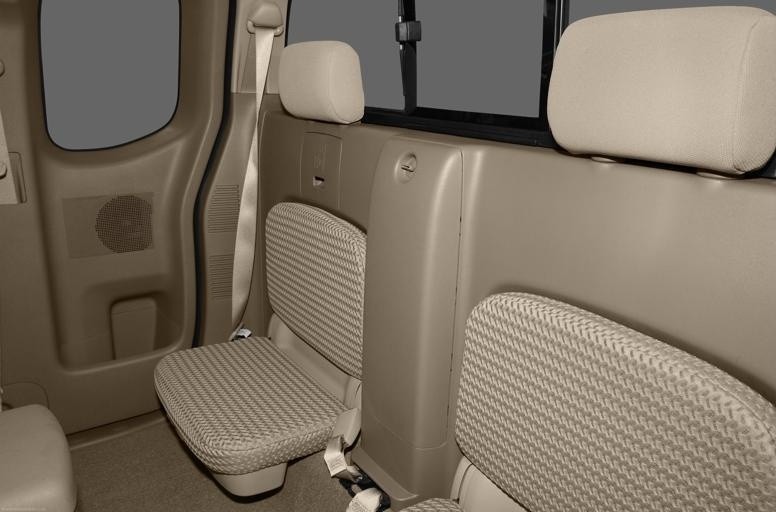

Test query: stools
[0,402,78,512]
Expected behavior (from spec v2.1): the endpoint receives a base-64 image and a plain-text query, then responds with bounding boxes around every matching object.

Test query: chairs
[151,41,369,500]
[403,5,776,510]
